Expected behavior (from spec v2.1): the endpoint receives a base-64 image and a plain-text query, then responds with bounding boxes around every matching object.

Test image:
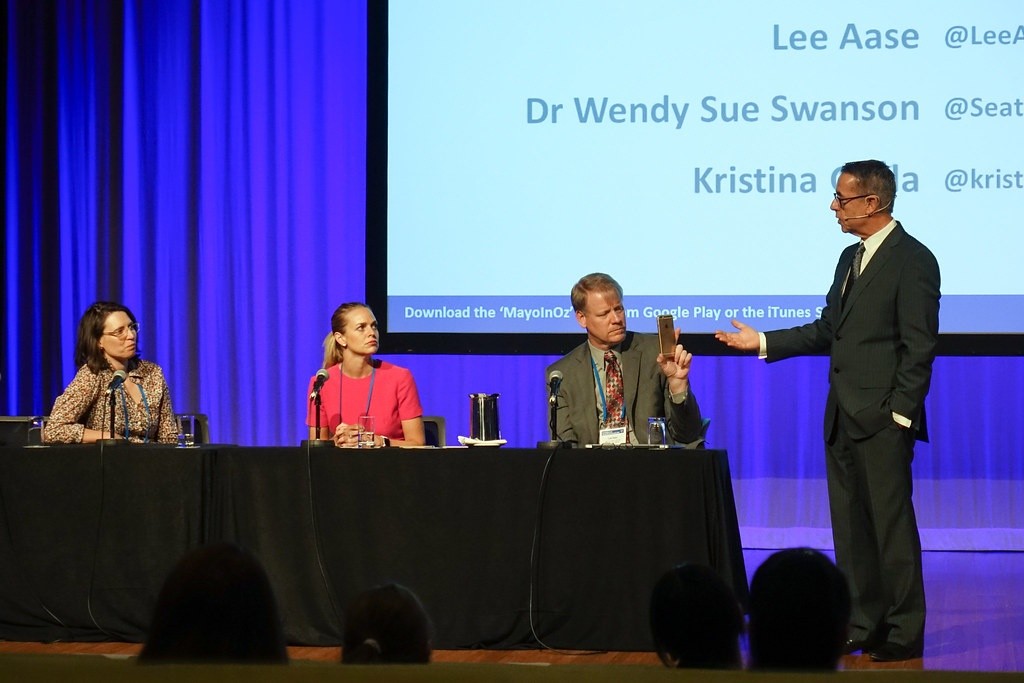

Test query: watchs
[381,436,391,448]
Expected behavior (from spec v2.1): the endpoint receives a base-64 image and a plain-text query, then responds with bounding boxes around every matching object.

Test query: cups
[648,417,668,450]
[176,416,195,447]
[468,392,500,441]
[358,416,376,448]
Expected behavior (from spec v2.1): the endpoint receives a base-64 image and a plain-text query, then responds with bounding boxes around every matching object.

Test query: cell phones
[657,315,677,357]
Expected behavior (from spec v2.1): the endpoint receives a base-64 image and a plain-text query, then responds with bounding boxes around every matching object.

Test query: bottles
[27,417,45,446]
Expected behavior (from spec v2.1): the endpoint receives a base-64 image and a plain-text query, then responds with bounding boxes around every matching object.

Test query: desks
[0,442,748,650]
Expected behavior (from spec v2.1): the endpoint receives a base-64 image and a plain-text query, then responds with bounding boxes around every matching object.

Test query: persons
[338,580,433,665]
[544,272,704,447]
[745,546,854,673]
[644,563,746,671]
[43,301,180,445]
[716,155,941,660]
[136,542,293,667]
[305,302,426,448]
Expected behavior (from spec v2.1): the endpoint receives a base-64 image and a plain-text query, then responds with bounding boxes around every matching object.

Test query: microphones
[549,371,563,404]
[843,212,875,220]
[310,368,330,401]
[105,370,126,397]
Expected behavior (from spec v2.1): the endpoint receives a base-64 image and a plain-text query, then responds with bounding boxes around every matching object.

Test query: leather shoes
[838,640,882,655]
[868,647,924,662]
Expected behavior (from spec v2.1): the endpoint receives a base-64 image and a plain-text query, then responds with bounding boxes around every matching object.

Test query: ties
[841,243,866,314]
[603,350,624,429]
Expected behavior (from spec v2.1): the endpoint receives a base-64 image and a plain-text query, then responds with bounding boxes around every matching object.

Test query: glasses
[102,322,140,338]
[833,192,877,209]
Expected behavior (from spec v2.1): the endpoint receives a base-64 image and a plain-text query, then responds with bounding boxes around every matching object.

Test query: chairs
[422,415,446,447]
[175,413,210,444]
